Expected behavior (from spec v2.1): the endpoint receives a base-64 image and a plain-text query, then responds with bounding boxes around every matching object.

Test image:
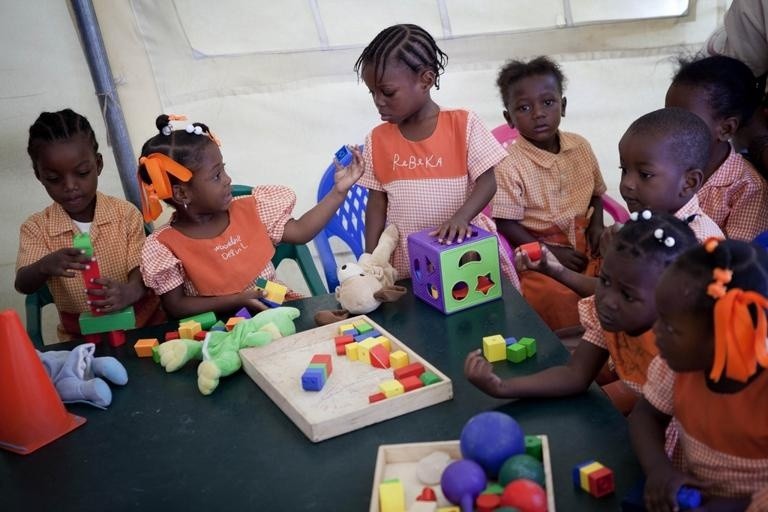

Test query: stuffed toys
[314,224,406,326]
[153,307,301,396]
[35,343,129,409]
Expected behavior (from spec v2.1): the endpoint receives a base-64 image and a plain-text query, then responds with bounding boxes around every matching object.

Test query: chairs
[480,123,630,275]
[25,219,154,349]
[314,145,372,293]
[227,182,327,297]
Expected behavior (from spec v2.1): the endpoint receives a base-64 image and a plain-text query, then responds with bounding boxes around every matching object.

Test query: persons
[354,24,523,298]
[464,207,702,402]
[707,0,768,182]
[15,108,165,343]
[137,114,365,323]
[513,108,727,417]
[599,54,768,259]
[489,56,607,357]
[626,237,768,512]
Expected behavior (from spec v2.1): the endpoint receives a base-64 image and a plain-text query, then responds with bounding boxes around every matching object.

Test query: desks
[0,269,646,512]
